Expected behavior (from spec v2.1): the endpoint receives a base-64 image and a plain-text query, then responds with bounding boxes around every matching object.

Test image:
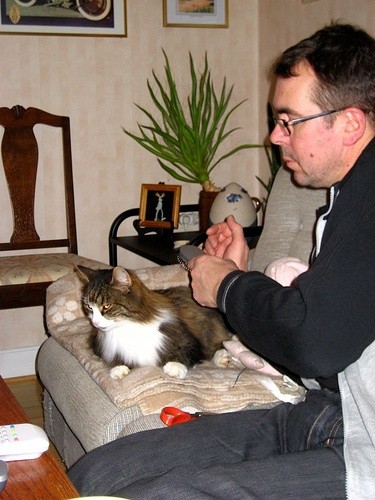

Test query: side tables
[109,198,267,270]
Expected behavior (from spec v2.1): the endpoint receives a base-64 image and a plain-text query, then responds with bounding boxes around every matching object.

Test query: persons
[64,15,375,500]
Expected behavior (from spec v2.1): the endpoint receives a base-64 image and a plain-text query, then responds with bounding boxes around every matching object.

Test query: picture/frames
[162,0,230,29]
[0,0,128,39]
[138,182,182,229]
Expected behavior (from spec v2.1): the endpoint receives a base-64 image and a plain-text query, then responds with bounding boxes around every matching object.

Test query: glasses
[272,107,365,136]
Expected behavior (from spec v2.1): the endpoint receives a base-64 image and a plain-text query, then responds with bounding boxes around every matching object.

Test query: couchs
[35,162,333,472]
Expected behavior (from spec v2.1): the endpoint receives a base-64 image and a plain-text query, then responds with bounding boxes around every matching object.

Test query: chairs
[0,104,115,309]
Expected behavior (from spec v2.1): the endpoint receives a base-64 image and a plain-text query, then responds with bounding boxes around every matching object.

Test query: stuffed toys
[222,257,310,376]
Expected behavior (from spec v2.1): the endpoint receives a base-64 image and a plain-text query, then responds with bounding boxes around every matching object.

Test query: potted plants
[118,45,275,234]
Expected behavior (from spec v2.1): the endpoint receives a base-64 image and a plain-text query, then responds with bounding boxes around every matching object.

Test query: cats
[75,264,233,380]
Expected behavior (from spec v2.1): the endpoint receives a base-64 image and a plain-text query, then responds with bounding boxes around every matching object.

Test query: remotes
[0,423,49,461]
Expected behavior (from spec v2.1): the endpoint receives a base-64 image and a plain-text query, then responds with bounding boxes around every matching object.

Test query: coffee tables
[0,372,82,500]
